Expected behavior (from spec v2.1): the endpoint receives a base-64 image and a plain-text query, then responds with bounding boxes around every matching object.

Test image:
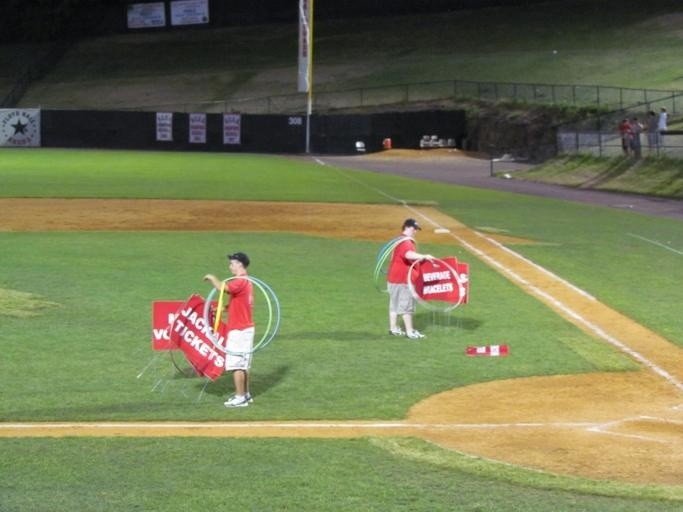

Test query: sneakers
[389,327,425,339]
[224,392,253,407]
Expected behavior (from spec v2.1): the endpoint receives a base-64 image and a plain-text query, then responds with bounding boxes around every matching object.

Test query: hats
[227,252,250,268]
[404,219,422,231]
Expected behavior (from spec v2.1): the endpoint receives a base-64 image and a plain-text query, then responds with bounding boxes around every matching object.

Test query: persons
[658,107,667,147]
[632,118,643,160]
[386,218,437,339]
[204,252,255,408]
[647,111,659,148]
[621,119,635,155]
[383,135,391,149]
